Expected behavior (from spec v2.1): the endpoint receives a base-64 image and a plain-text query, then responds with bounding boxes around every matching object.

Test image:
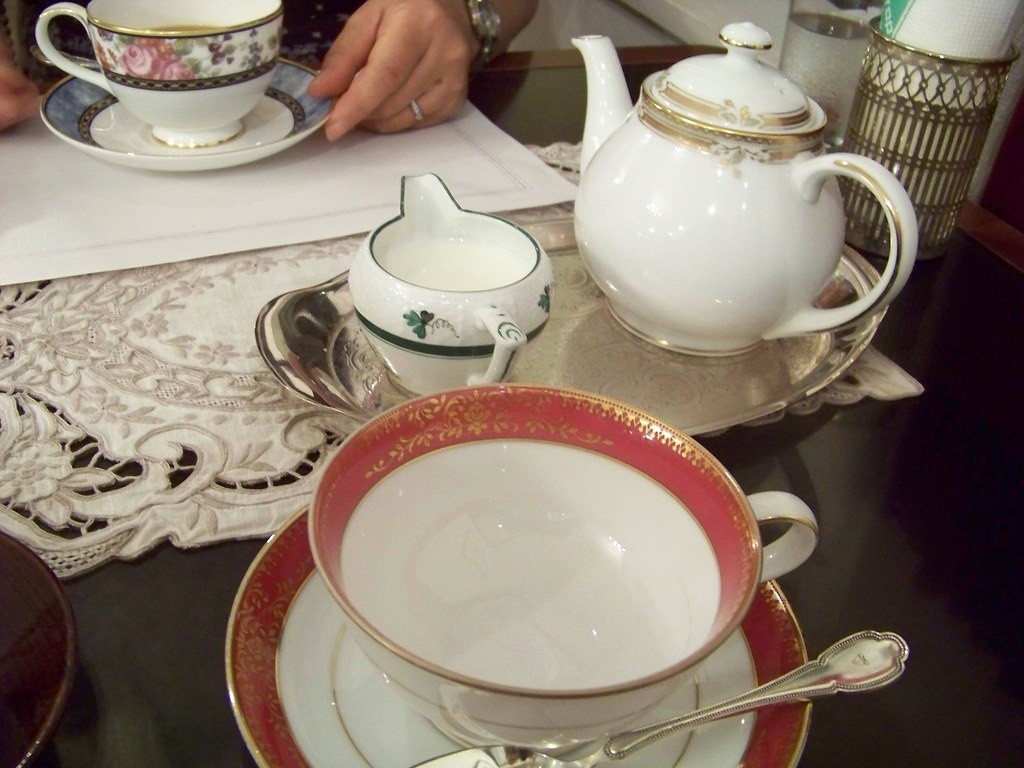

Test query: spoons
[411,632,908,768]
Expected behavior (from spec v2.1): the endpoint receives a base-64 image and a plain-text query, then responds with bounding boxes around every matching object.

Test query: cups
[778,0,883,147]
[35,0,284,145]
[307,384,818,748]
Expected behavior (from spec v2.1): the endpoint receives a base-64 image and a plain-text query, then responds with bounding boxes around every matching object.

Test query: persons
[0,0,535,143]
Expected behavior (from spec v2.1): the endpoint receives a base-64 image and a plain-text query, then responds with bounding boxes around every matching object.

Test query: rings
[412,100,423,122]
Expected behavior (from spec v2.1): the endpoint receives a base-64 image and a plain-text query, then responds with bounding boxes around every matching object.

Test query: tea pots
[572,26,920,357]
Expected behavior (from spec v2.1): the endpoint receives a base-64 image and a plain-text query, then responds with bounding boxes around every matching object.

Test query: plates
[40,54,337,171]
[224,506,812,768]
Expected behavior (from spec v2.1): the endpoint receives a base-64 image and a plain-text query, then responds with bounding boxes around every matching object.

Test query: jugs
[350,174,554,397]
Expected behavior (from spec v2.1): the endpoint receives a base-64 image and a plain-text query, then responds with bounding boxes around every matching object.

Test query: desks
[0,144,1024,768]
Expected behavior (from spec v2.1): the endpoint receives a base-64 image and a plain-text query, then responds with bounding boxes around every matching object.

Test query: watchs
[464,1,502,66]
